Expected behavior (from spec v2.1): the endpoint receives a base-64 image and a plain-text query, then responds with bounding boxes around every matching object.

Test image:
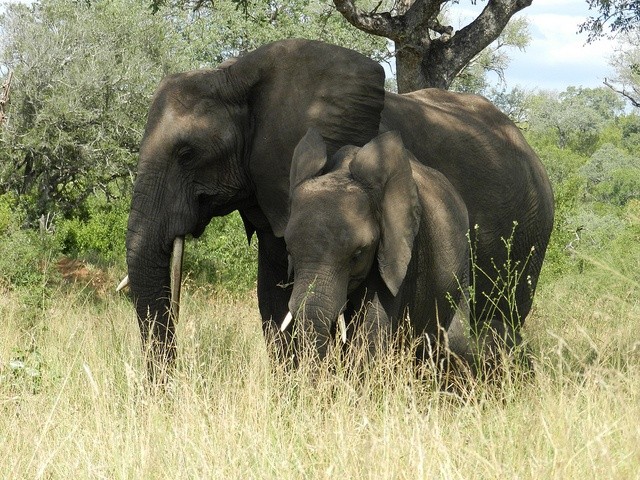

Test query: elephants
[280,124,474,398]
[114,35,558,399]
